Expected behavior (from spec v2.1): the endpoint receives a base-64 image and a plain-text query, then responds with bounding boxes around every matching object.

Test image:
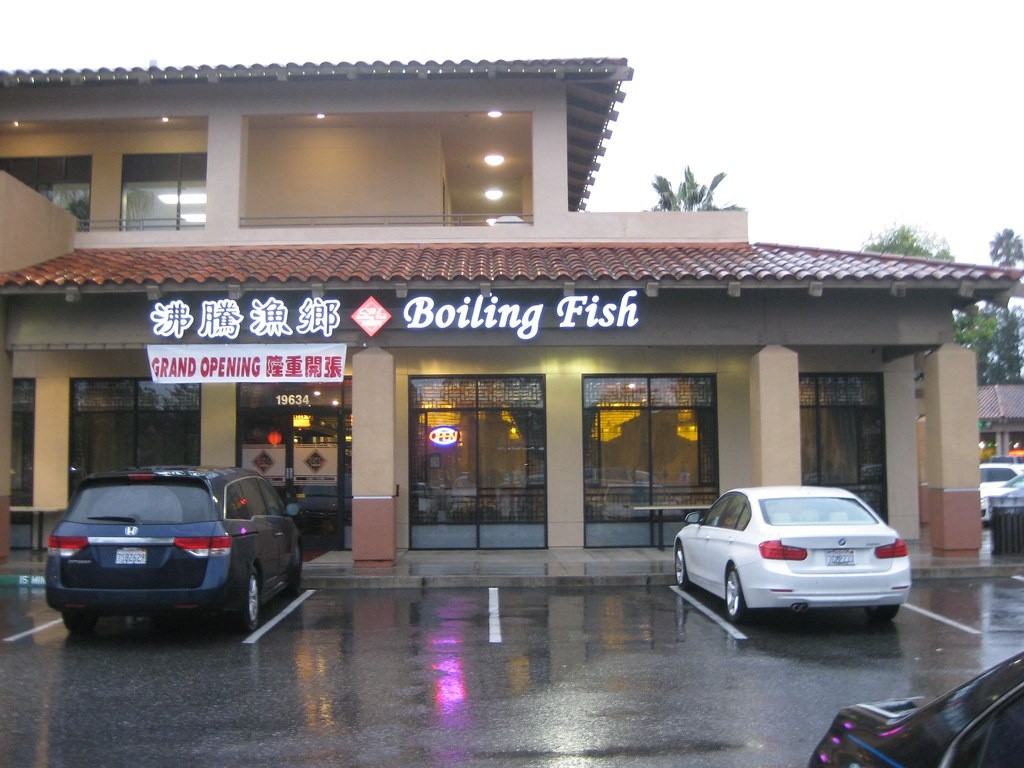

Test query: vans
[44,467,303,632]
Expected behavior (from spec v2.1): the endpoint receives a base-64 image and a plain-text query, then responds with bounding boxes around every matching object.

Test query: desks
[448,489,498,511]
[10,505,66,556]
[625,504,711,550]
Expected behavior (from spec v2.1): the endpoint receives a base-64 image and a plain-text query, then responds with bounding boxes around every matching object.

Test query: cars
[674,486,911,624]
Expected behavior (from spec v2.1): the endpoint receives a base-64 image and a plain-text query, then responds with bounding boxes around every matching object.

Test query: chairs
[419,468,691,518]
